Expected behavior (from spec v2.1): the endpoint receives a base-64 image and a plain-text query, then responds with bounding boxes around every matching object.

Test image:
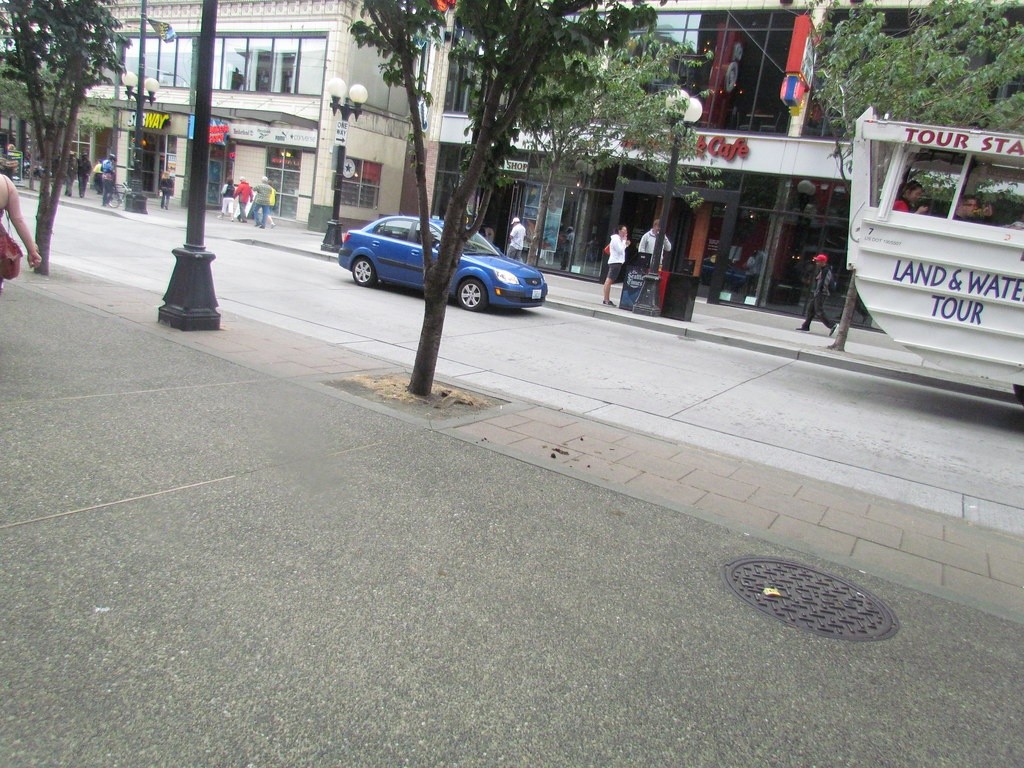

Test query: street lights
[783,179,817,303]
[560,160,596,270]
[320,77,369,253]
[123,71,161,214]
[631,90,706,316]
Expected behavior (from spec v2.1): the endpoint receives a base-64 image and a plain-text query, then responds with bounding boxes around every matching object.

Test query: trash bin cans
[619,251,652,311]
[648,269,670,311]
[660,258,700,322]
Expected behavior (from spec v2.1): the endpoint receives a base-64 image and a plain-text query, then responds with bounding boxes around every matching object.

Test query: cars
[22,159,53,180]
[336,216,548,313]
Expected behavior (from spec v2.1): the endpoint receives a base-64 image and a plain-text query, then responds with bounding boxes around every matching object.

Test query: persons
[217,179,235,220]
[835,286,869,324]
[535,187,564,251]
[65,151,78,197]
[950,193,993,225]
[76,153,92,198]
[602,225,631,307]
[253,175,278,229]
[506,217,527,264]
[892,180,928,214]
[559,226,577,271]
[742,248,775,301]
[796,253,839,336]
[158,172,174,209]
[93,154,117,208]
[233,176,253,223]
[638,219,672,275]
[0,174,42,299]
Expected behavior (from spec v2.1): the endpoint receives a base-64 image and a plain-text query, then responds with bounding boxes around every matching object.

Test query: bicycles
[106,181,130,208]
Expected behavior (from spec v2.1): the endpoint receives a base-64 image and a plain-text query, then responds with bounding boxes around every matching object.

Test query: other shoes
[796,327,809,332]
[241,220,247,223]
[237,216,240,221]
[260,226,265,229]
[165,205,168,210]
[603,301,616,307]
[255,224,260,226]
[829,324,837,336]
[271,224,276,228]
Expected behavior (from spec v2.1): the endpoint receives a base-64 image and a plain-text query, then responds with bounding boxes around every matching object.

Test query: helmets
[110,154,116,160]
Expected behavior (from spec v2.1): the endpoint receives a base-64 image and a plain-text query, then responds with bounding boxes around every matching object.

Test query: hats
[567,227,573,232]
[511,217,520,224]
[813,255,827,263]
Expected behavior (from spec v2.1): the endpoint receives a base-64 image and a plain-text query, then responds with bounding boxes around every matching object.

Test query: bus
[844,106,1024,407]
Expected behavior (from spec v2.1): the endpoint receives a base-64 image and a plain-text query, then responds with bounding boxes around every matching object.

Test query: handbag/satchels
[605,244,610,255]
[4,233,23,279]
[221,184,229,194]
